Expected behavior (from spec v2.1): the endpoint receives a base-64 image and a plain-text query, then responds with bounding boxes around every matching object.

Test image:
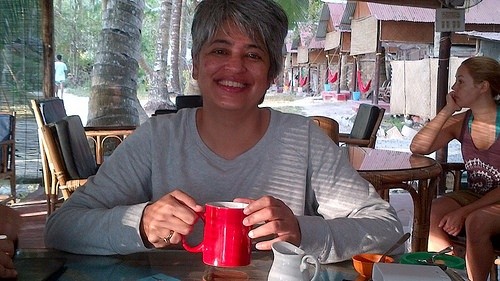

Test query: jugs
[268,241,321,281]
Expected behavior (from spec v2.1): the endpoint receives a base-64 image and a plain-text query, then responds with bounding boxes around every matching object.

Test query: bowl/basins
[352,254,394,277]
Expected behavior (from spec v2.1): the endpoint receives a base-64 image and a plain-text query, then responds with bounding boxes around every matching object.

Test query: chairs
[0,97,386,222]
[437,162,500,267]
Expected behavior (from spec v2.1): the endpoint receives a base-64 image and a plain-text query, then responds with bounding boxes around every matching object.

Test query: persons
[43,0,404,264]
[55,54,67,99]
[410,56,500,281]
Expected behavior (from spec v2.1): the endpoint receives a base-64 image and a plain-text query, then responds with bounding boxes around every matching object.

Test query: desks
[83,126,136,164]
[0,250,404,281]
[338,147,443,252]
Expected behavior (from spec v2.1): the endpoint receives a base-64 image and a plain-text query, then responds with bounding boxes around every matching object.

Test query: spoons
[415,246,454,263]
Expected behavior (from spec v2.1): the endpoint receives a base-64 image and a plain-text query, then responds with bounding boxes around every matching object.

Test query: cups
[182,202,253,268]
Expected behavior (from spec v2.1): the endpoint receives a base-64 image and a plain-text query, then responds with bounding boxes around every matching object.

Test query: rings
[163,229,174,244]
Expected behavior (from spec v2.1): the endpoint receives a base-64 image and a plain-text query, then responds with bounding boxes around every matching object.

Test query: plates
[399,252,466,271]
